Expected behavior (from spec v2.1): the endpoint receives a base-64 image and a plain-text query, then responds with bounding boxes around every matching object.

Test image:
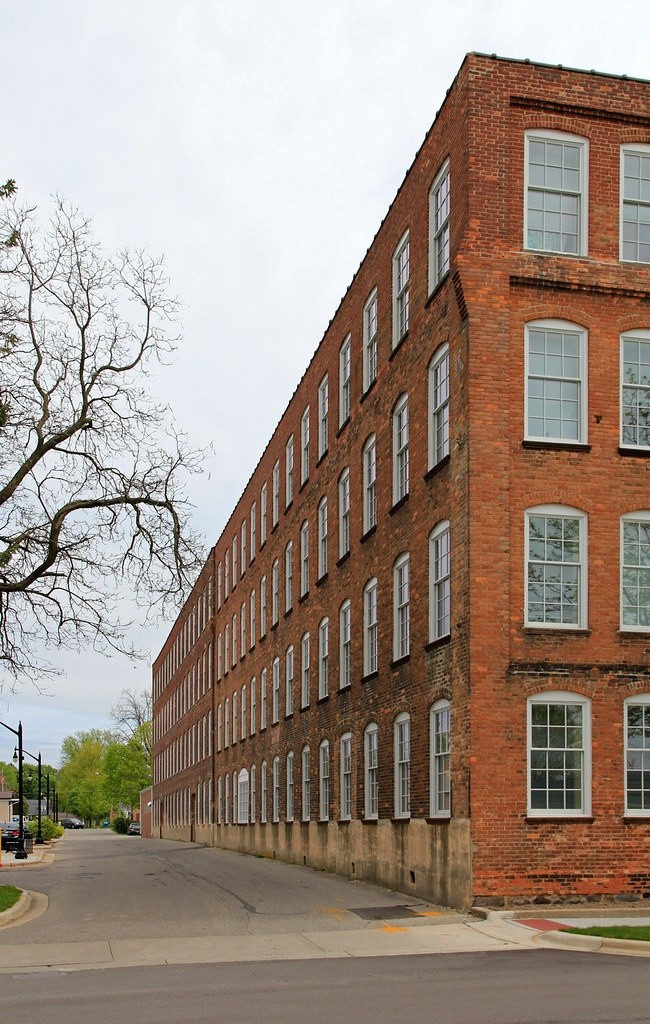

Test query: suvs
[61,817,84,830]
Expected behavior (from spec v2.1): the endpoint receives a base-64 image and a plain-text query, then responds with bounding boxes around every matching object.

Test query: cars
[126,823,141,836]
[0,813,28,850]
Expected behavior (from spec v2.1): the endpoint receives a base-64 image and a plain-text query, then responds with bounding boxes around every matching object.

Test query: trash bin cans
[26,832,34,854]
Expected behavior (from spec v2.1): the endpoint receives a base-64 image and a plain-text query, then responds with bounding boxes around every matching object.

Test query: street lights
[13,746,45,845]
[40,787,56,824]
[28,768,50,818]
